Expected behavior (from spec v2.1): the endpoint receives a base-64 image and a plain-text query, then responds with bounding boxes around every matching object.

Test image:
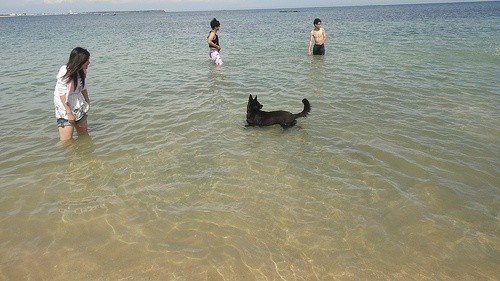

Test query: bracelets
[67,111,72,114]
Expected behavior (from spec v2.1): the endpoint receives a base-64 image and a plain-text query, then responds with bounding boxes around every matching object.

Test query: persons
[207,18,223,65]
[53,47,90,142]
[308,18,326,55]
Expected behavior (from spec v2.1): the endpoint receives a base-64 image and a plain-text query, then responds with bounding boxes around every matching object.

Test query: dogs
[244,94,312,130]
